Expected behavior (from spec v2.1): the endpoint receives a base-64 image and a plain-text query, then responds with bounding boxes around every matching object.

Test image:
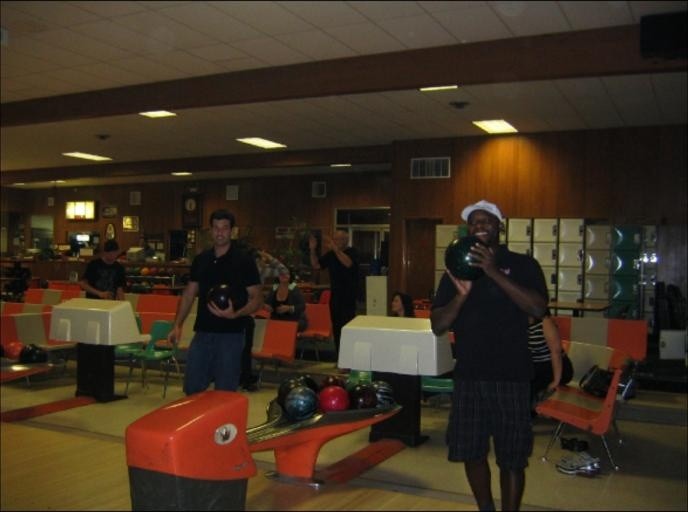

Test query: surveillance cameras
[99,134,109,139]
[450,101,469,109]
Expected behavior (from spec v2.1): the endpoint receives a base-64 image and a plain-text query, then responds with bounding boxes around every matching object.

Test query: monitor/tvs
[127,247,145,261]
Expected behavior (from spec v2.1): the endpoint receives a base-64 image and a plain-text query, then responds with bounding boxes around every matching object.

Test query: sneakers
[238,374,259,392]
[555,438,601,478]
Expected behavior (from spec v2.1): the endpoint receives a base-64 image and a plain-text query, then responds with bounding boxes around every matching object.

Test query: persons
[166,208,265,397]
[392,290,418,319]
[139,237,154,257]
[429,200,550,512]
[262,267,308,333]
[238,296,260,392]
[526,310,574,411]
[309,230,360,374]
[77,238,127,301]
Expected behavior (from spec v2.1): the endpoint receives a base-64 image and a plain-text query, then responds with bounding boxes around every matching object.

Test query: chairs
[1,282,335,400]
[536,317,650,473]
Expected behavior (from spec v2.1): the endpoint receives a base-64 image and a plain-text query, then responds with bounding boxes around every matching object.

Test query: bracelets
[290,304,297,314]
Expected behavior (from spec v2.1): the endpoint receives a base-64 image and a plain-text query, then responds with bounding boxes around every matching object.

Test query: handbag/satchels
[580,365,613,398]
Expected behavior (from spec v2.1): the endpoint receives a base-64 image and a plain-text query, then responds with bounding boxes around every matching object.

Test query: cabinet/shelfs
[434,218,658,334]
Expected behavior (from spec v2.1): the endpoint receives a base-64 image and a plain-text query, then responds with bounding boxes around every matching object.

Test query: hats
[460,200,504,223]
